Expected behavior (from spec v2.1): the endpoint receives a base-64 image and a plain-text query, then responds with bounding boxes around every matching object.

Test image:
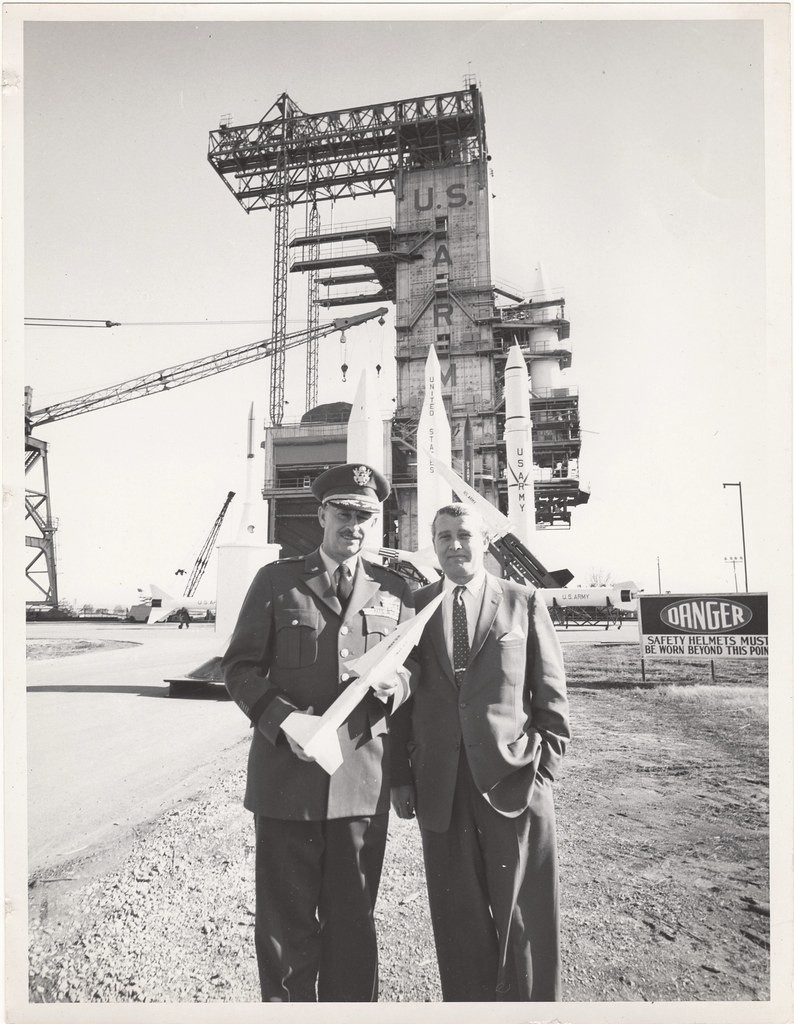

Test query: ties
[337,564,353,614]
[452,586,471,690]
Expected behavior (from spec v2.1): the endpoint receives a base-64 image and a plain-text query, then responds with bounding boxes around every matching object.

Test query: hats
[311,463,390,513]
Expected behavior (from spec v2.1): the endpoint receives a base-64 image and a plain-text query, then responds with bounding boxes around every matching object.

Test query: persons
[221,465,417,1003]
[391,504,570,1002]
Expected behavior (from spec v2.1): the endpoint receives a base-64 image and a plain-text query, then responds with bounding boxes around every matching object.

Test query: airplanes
[280,585,449,776]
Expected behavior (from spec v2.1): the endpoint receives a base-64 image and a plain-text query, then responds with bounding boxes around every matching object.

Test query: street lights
[723,556,744,591]
[724,483,748,592]
